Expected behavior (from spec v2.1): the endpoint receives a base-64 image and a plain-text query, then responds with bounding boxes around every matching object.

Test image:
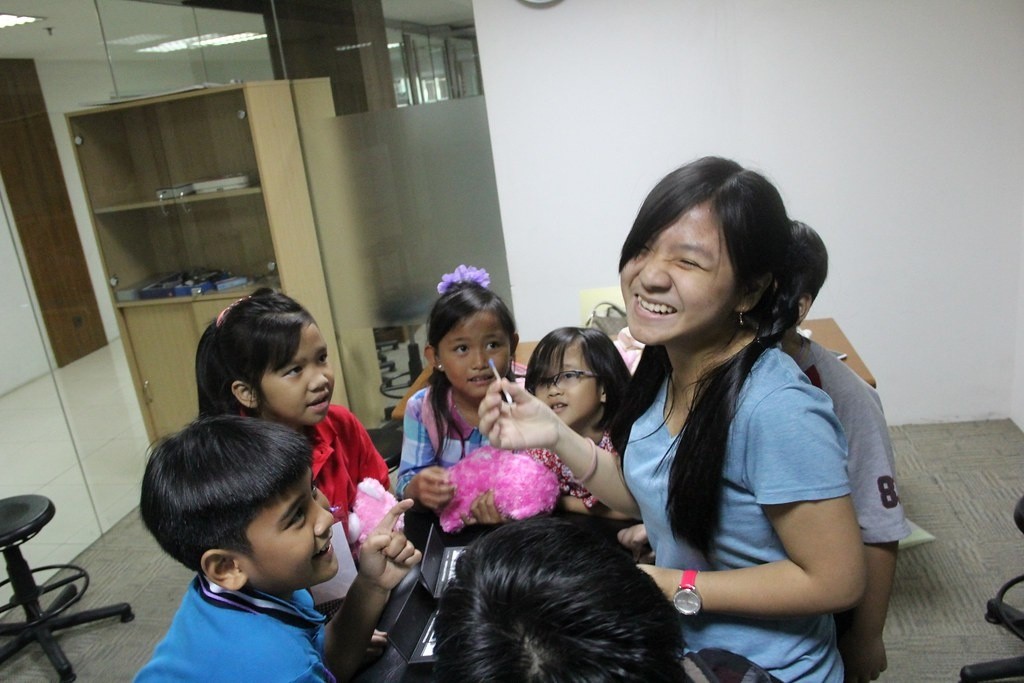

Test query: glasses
[528,370,599,392]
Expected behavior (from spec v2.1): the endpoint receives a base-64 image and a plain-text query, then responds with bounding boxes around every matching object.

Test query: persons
[617,220,912,683]
[194,287,397,661]
[129,416,424,683]
[395,265,519,527]
[524,327,637,523]
[435,518,787,683]
[477,157,866,683]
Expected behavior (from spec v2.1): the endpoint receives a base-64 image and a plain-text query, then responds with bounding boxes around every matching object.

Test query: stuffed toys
[430,440,559,538]
[348,477,408,578]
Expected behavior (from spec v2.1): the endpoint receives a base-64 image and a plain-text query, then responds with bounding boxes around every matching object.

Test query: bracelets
[571,436,597,484]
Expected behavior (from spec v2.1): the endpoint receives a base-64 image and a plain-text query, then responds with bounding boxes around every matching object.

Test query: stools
[0,494,135,683]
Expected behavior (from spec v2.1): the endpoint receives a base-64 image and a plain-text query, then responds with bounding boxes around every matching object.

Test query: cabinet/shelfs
[62,80,352,452]
[288,73,392,429]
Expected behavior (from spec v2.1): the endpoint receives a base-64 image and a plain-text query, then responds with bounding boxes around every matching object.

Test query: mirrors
[0,1,281,614]
[269,0,516,431]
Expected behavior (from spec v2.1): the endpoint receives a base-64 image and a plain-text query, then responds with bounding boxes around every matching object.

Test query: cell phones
[829,350,847,360]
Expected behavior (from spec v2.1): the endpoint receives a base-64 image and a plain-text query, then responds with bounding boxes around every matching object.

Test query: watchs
[673,568,702,620]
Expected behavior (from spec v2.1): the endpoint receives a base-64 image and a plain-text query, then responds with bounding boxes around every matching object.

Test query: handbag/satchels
[585,302,629,341]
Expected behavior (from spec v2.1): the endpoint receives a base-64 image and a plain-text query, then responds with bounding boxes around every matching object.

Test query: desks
[389,314,876,421]
